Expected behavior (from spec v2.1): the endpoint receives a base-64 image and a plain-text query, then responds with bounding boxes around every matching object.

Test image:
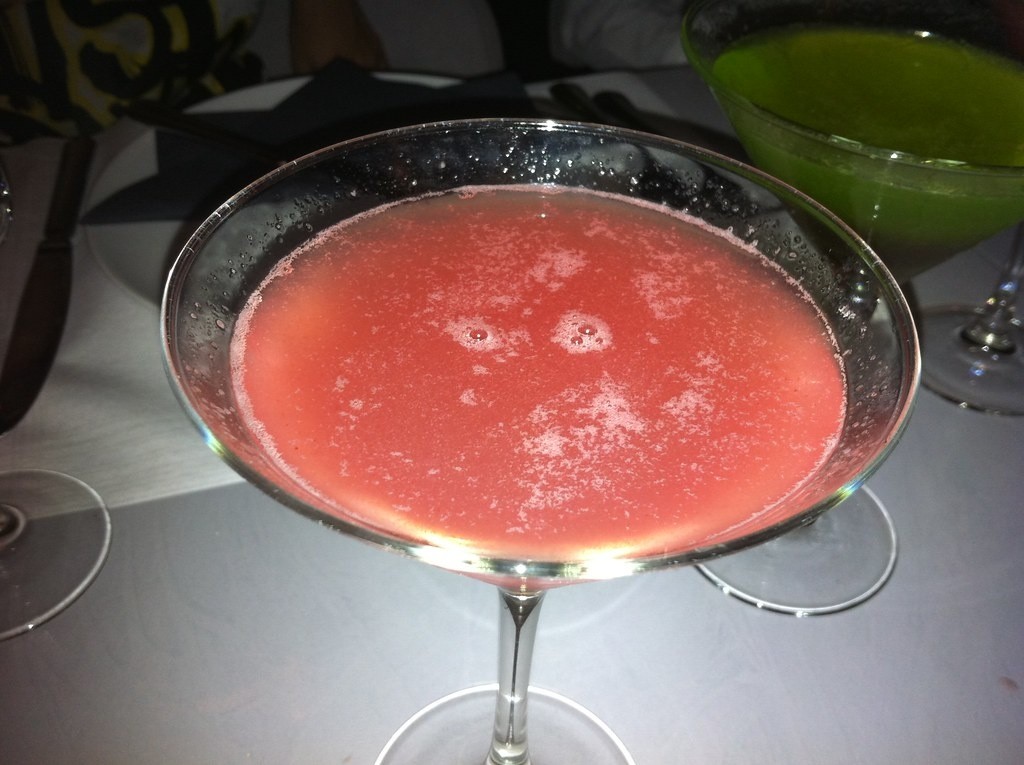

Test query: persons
[223,0,689,74]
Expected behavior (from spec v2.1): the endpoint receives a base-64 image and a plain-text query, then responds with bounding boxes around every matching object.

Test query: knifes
[1,130,99,431]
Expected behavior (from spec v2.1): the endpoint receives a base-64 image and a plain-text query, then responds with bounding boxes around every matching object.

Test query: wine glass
[916,222,1024,416]
[680,0,1024,617]
[160,117,922,765]
[0,176,112,642]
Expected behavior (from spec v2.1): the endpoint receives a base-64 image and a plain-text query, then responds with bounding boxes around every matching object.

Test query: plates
[80,68,465,307]
